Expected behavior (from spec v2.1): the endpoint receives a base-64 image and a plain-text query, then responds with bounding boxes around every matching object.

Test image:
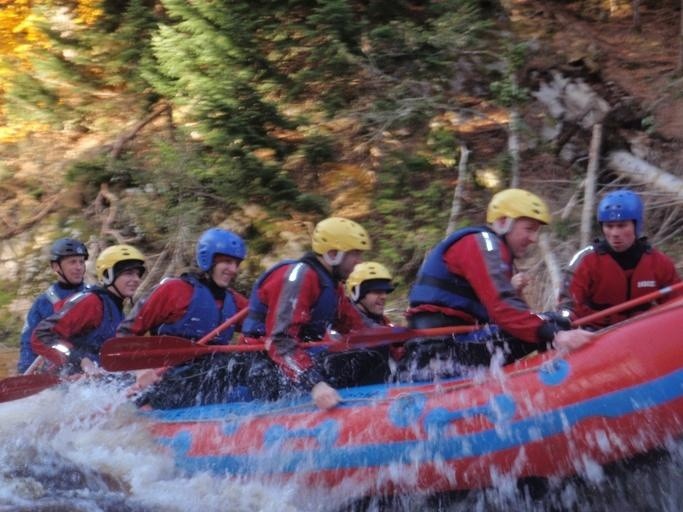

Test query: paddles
[0,375,104,402]
[102,335,350,372]
[349,325,479,348]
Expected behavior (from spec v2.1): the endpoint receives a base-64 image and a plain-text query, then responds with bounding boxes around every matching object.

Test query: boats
[0,283,682,511]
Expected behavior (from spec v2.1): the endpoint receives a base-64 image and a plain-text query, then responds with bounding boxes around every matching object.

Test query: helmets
[597,191,643,239]
[196,229,246,271]
[50,239,87,263]
[312,217,374,265]
[95,245,147,287]
[346,261,394,302]
[488,189,549,225]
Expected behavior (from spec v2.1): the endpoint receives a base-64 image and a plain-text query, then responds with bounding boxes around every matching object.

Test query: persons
[333,259,406,387]
[404,187,568,379]
[31,242,150,377]
[225,218,395,411]
[115,230,253,413]
[554,190,683,335]
[15,238,89,377]
